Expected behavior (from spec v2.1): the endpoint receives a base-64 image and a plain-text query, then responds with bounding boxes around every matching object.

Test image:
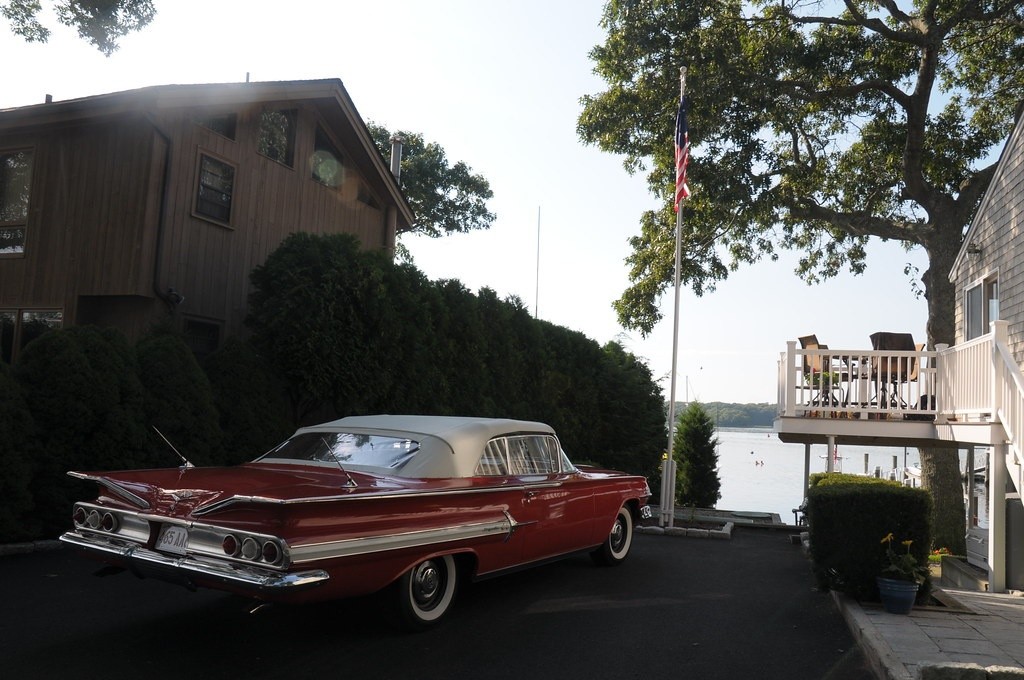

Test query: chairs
[798,335,859,407]
[870,332,926,409]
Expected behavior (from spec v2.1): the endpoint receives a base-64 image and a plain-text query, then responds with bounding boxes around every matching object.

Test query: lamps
[965,242,983,261]
[168,287,185,305]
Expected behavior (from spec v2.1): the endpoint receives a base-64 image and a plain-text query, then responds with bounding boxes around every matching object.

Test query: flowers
[881,532,931,585]
[803,365,839,386]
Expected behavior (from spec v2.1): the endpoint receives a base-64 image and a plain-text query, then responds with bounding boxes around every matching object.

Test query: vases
[874,576,920,614]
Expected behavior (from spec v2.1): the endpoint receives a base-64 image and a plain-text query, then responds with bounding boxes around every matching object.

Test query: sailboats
[666,375,724,445]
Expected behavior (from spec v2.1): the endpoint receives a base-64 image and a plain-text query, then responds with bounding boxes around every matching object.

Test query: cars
[56,415,656,627]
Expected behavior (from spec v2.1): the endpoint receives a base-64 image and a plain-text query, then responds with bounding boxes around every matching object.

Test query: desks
[832,356,878,408]
